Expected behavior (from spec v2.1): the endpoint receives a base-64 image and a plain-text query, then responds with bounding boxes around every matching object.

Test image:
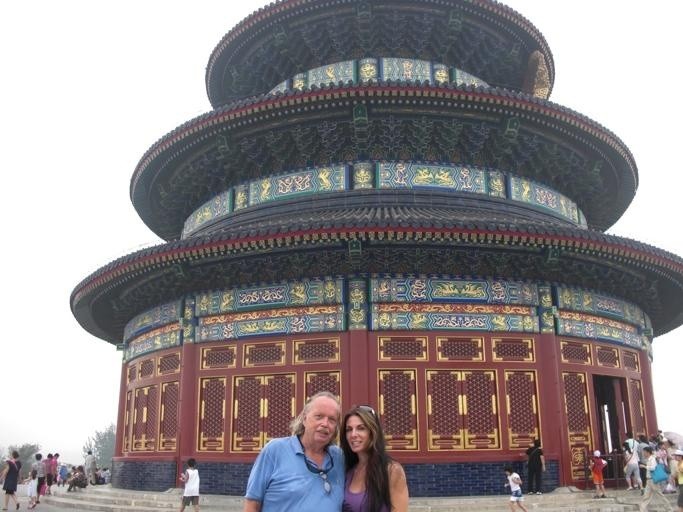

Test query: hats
[671,449,683,458]
[594,450,600,456]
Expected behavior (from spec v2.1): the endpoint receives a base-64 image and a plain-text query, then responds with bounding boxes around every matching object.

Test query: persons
[0,450,111,511]
[503,466,528,512]
[622,430,683,511]
[519,439,545,495]
[244,390,345,512]
[337,403,409,512]
[178,457,200,511]
[587,449,608,498]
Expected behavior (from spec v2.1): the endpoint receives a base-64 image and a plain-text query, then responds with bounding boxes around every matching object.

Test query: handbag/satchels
[623,466,629,472]
[523,462,530,474]
[650,464,668,484]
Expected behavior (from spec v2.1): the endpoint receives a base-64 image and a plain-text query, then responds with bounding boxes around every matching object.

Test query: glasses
[306,454,335,474]
[319,469,331,493]
[357,405,376,417]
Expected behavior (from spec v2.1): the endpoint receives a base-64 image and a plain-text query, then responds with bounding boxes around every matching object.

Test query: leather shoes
[16,503,19,510]
[1,508,7,510]
[28,499,41,508]
